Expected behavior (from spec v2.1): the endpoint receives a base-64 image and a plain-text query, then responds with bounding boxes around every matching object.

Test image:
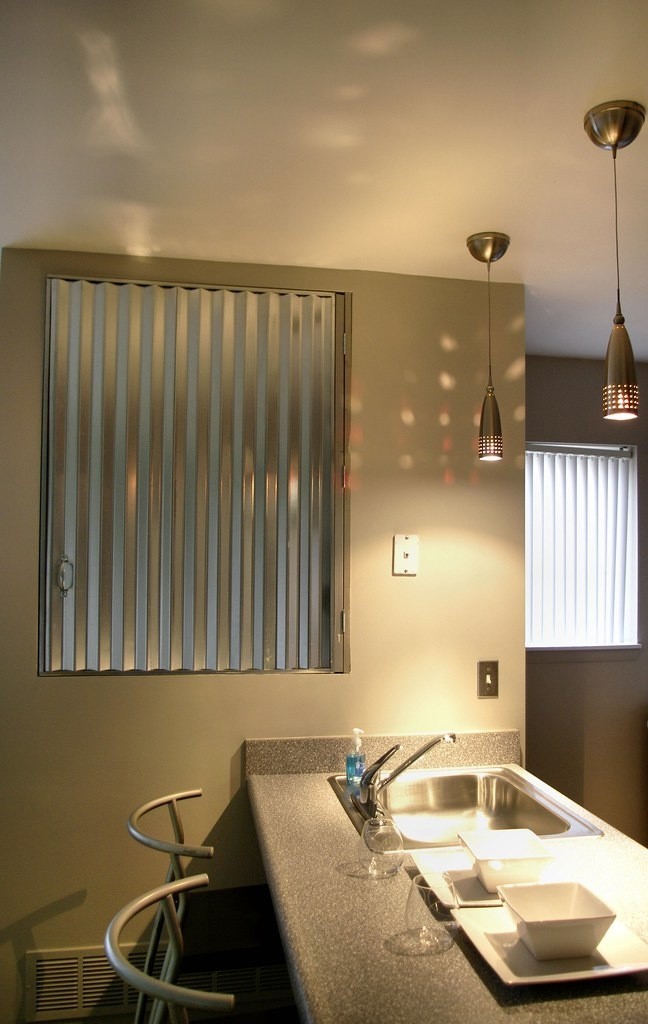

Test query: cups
[357,818,404,878]
[404,872,460,947]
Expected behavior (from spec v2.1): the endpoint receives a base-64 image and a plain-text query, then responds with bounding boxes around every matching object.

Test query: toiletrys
[346,728,366,787]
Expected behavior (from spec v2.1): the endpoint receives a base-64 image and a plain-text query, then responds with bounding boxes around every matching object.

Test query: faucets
[360,733,457,804]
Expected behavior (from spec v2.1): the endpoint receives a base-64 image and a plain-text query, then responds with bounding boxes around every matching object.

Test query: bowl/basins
[496,881,616,961]
[456,828,553,893]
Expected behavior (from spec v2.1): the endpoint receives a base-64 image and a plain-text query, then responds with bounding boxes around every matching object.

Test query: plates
[450,907,648,986]
[411,850,554,908]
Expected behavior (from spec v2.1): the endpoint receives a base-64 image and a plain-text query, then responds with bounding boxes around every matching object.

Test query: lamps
[582,99,646,422]
[466,231,511,463]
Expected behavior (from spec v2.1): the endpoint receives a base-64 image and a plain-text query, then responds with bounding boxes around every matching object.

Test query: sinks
[327,767,604,850]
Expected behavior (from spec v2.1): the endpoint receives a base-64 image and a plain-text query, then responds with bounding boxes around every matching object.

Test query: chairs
[102,790,307,1024]
